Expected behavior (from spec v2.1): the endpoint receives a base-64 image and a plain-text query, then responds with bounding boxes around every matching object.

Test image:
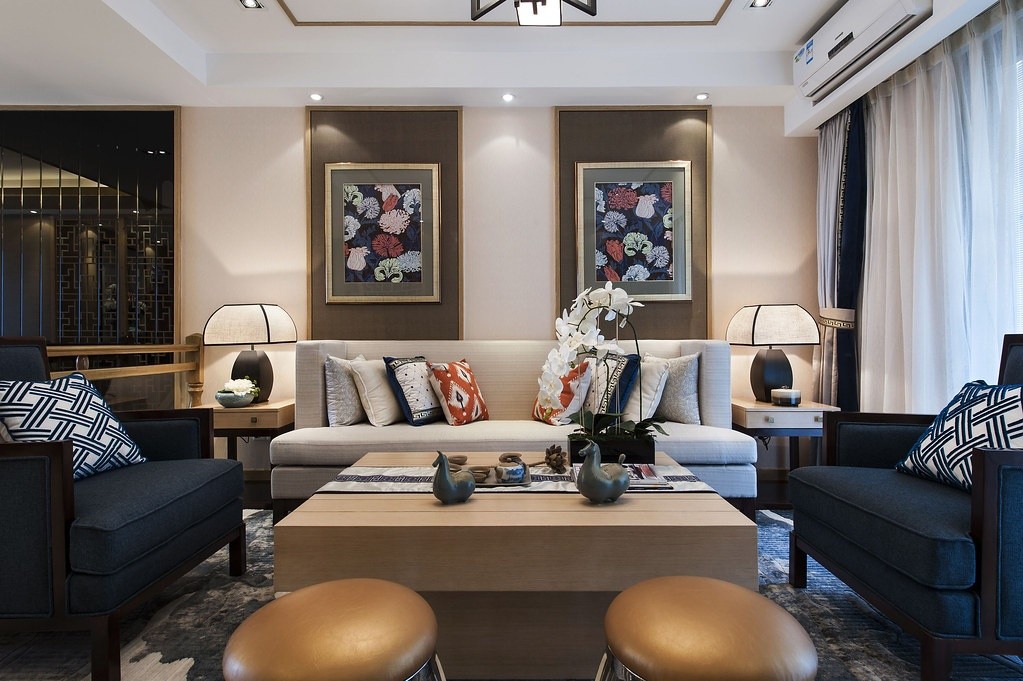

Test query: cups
[449,465,463,472]
[469,466,490,476]
[448,455,467,465]
[471,473,486,483]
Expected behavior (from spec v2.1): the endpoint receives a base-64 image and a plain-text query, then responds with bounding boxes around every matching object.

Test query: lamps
[726,303,820,401]
[470,0,598,26]
[203,304,298,402]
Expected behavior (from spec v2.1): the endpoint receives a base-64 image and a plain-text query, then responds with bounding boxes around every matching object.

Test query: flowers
[219,376,260,397]
[536,280,671,442]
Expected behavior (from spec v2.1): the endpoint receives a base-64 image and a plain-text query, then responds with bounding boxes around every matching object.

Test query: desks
[192,397,295,460]
[732,396,842,472]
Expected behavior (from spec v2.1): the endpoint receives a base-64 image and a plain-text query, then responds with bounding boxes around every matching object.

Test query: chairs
[0,336,247,681]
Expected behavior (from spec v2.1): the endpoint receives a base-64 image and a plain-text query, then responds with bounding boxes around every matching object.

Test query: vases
[215,390,254,408]
[567,435,656,466]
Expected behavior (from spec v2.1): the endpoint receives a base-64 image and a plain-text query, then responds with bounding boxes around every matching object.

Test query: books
[570,463,675,491]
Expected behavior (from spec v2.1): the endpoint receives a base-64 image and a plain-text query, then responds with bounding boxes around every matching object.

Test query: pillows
[531,352,703,427]
[322,354,489,427]
[897,381,1023,490]
[0,371,146,479]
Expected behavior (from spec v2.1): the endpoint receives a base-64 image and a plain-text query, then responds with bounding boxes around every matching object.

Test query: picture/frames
[325,164,442,303]
[574,161,693,303]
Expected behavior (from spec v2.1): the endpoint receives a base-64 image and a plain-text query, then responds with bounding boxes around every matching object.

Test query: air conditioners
[792,0,932,105]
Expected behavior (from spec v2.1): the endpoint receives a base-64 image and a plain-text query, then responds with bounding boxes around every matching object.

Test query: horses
[431,450,477,505]
[576,438,630,504]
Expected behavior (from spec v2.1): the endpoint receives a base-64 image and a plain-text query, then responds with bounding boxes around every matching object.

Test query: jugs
[494,452,526,484]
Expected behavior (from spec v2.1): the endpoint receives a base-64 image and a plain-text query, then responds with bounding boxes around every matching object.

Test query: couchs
[788,332,1022,681]
[267,338,758,523]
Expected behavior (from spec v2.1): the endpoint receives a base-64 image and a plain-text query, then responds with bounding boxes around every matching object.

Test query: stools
[222,577,447,681]
[594,577,819,681]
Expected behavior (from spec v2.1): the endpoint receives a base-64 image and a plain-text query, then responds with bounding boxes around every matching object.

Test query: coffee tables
[274,451,760,680]
